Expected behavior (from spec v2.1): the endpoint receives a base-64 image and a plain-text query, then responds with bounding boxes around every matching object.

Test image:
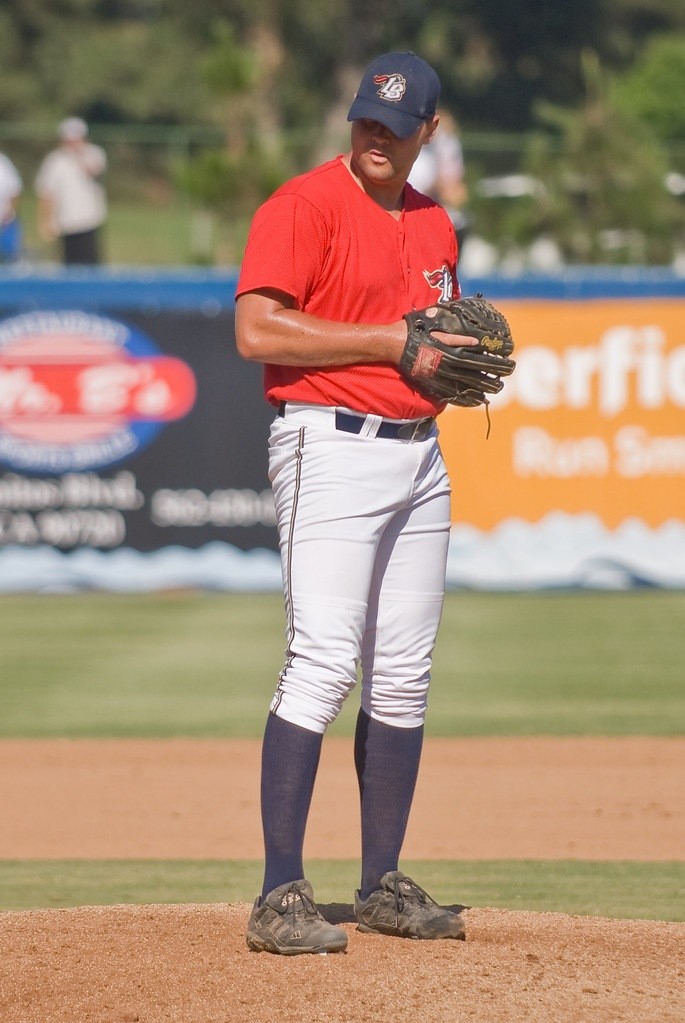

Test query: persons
[0,151,24,264]
[404,117,472,255]
[236,49,516,956]
[32,117,109,265]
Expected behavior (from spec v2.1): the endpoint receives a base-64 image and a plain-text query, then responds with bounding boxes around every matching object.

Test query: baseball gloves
[396,295,517,408]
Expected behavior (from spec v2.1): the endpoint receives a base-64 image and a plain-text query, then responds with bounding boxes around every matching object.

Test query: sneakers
[353,872,467,944]
[246,879,348,956]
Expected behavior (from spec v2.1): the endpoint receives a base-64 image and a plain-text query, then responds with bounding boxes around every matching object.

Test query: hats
[347,50,441,141]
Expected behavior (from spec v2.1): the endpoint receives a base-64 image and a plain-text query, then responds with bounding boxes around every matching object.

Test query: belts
[276,400,436,443]
